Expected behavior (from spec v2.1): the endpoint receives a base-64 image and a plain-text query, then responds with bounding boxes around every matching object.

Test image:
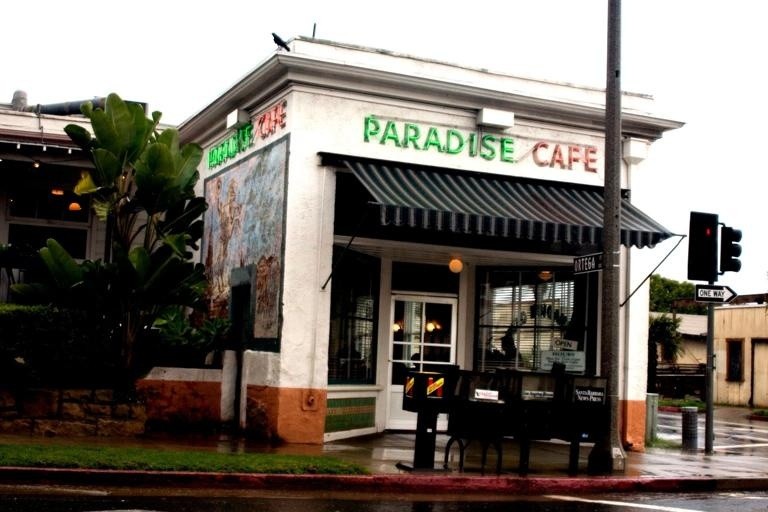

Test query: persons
[499,335,523,367]
[411,353,430,371]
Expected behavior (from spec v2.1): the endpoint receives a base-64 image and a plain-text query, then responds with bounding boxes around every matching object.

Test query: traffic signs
[695,285,737,303]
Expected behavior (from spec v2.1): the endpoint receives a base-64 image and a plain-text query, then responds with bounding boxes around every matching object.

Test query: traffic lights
[687,212,742,282]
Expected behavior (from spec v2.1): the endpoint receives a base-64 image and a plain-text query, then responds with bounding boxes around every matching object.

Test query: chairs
[444,369,612,479]
